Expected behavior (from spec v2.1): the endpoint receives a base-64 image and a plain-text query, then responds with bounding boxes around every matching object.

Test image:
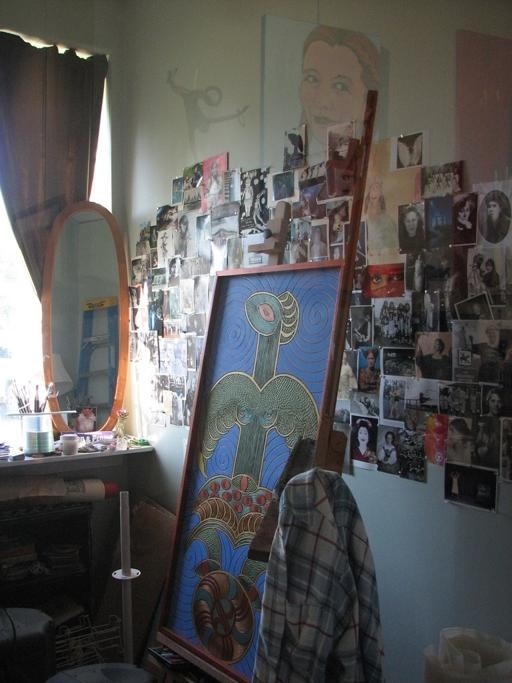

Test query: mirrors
[39,200,132,434]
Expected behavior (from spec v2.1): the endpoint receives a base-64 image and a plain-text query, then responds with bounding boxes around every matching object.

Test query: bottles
[115,417,127,450]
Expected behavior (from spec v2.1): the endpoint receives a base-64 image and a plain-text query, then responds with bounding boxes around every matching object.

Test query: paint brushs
[12,378,55,413]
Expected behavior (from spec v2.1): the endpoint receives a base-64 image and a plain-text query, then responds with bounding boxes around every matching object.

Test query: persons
[242,178,255,217]
[205,160,223,210]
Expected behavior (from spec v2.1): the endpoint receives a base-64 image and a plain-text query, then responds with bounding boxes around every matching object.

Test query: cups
[63,435,78,457]
[22,414,56,455]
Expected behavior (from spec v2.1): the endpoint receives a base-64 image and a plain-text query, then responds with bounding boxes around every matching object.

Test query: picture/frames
[155,257,354,675]
